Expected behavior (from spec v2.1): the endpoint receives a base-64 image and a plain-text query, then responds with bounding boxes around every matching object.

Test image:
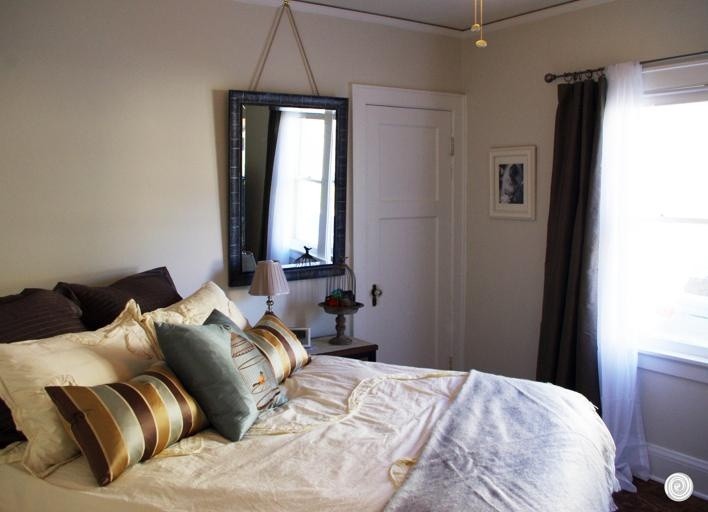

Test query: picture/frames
[289,328,311,348]
[488,145,536,222]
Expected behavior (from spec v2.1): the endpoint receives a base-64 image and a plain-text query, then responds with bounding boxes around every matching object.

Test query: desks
[305,334,378,362]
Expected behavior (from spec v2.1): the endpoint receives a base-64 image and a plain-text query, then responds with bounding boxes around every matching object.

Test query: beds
[0,355,620,512]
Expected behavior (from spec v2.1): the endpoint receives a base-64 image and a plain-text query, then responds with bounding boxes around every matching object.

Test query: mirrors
[228,89,347,286]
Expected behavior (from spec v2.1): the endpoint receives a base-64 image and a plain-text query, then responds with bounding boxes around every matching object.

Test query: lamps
[248,260,294,314]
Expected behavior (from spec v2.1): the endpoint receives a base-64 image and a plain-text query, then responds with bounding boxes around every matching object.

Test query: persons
[504,163,519,204]
